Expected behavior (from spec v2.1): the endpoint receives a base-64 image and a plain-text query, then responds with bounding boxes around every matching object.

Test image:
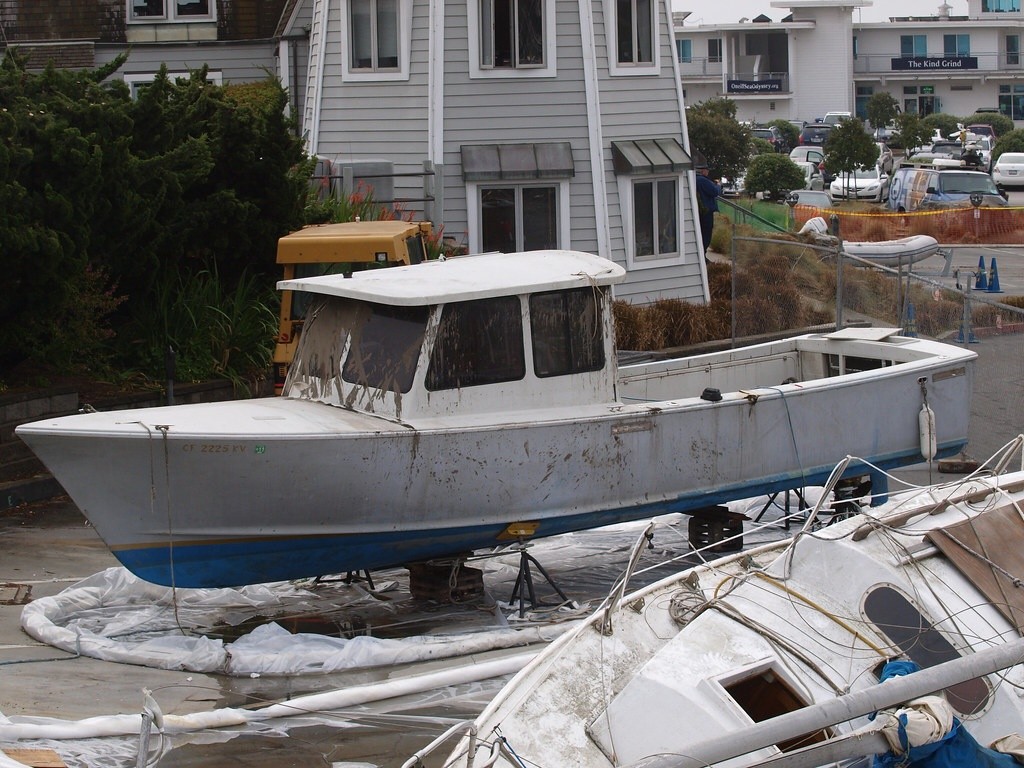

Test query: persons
[961,148,980,171]
[696,156,721,264]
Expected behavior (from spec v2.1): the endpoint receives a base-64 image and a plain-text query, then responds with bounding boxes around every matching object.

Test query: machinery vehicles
[270,218,442,397]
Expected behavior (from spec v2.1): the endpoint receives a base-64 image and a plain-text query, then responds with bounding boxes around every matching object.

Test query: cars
[830,163,891,206]
[715,111,998,200]
[783,191,841,211]
[991,152,1024,190]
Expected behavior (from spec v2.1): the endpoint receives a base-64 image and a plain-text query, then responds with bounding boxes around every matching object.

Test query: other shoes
[705,257,711,265]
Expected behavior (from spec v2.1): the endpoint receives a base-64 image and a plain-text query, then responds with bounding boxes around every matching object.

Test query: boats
[13,250,979,587]
[838,235,942,267]
[402,464,1024,768]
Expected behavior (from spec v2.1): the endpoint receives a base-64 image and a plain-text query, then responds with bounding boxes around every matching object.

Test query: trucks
[889,167,1009,213]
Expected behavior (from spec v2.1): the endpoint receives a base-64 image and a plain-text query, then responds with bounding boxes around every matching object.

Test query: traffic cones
[955,303,979,344]
[986,258,1005,293]
[904,304,921,338]
[972,256,988,290]
